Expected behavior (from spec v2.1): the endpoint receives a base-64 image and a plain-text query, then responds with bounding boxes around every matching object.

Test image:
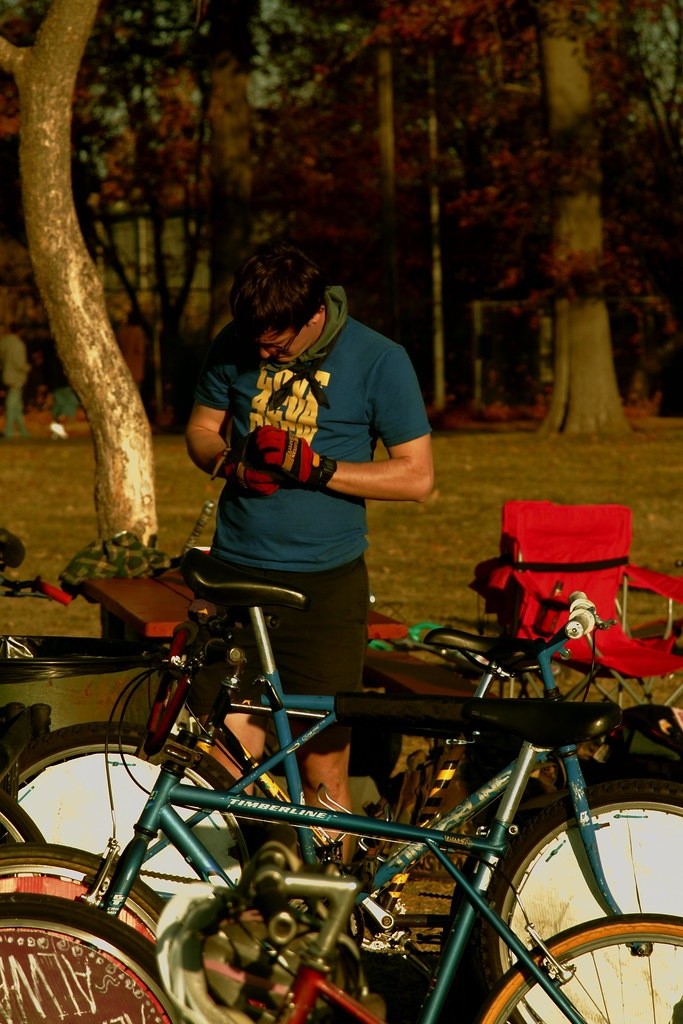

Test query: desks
[84,579,407,640]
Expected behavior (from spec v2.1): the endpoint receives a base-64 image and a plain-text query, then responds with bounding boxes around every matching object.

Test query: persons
[47,357,78,440]
[186,238,435,865]
[0,320,30,436]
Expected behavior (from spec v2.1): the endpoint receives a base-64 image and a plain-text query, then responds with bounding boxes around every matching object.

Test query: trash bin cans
[1,634,160,729]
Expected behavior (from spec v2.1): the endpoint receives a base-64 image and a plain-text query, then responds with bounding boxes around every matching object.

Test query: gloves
[208,447,283,496]
[248,425,323,489]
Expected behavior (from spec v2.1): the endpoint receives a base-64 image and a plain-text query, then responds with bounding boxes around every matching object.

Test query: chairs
[469,499,683,709]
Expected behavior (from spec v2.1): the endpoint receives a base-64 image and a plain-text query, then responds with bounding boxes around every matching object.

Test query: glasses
[235,326,304,355]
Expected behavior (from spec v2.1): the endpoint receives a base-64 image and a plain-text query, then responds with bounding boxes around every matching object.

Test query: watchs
[319,456,337,488]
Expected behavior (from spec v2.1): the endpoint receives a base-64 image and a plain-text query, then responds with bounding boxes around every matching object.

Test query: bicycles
[0,544,683,1024]
